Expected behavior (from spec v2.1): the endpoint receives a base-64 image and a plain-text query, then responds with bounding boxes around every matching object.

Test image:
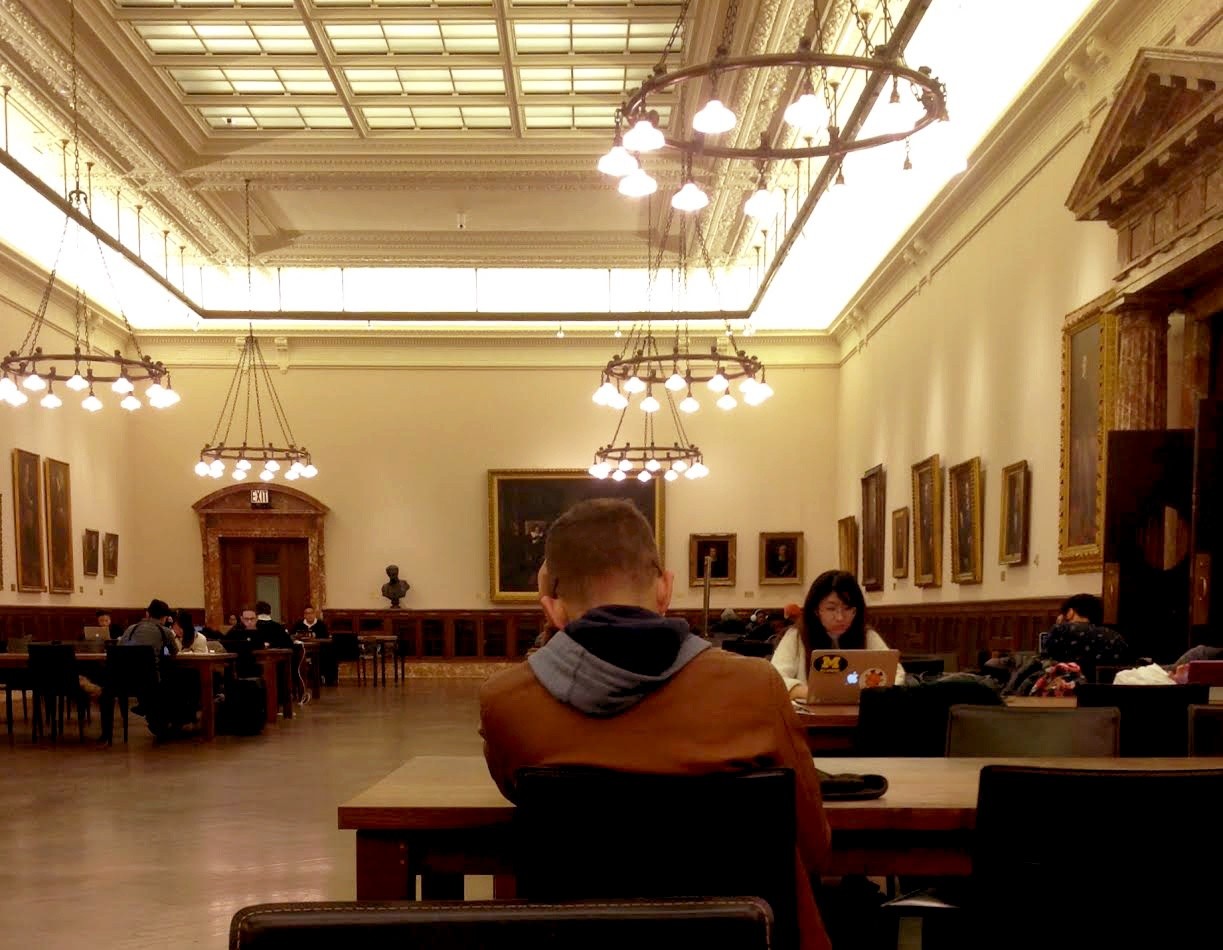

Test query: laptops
[83,625,114,641]
[792,646,901,707]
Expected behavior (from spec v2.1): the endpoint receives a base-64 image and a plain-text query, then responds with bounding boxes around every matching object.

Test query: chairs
[0,631,1223,950]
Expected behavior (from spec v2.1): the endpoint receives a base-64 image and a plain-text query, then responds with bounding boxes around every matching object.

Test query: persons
[1040,595,1136,683]
[479,499,833,950]
[771,571,905,896]
[79,599,338,741]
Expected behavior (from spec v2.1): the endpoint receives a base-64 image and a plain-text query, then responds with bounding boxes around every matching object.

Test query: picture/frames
[911,286,1116,586]
[487,468,665,604]
[838,516,856,579]
[861,463,884,586]
[892,506,908,577]
[690,532,737,587]
[758,531,804,585]
[0,448,119,595]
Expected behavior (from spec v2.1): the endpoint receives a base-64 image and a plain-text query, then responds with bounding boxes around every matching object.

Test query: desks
[355,634,405,689]
[0,653,236,740]
[790,697,1076,726]
[337,756,1223,903]
[255,648,293,722]
[291,637,332,700]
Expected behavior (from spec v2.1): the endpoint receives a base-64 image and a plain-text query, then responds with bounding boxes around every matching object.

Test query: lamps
[0,0,181,412]
[586,194,709,482]
[591,0,773,413]
[597,0,952,220]
[195,181,318,481]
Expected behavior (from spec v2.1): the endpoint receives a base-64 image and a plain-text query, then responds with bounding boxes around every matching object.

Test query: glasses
[818,604,856,615]
